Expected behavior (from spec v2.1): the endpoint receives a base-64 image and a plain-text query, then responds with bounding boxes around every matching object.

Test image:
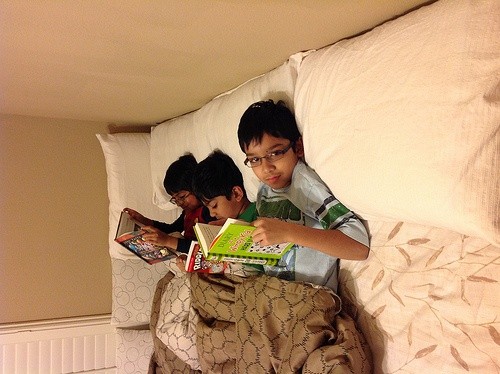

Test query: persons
[190,148,259,287]
[237,98,370,309]
[123,151,217,283]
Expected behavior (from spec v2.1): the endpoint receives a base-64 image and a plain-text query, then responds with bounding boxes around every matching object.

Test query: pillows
[115,328,154,374]
[294,0,500,248]
[95,132,180,261]
[150,48,316,210]
[110,257,169,328]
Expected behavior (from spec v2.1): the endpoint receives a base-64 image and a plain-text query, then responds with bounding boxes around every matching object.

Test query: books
[191,218,296,266]
[113,210,178,266]
[177,241,231,273]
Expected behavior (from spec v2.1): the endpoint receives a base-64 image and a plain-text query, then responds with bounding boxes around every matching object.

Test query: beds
[336,221,500,374]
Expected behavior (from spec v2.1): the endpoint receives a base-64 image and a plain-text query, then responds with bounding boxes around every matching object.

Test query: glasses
[244,141,294,168]
[169,193,190,204]
